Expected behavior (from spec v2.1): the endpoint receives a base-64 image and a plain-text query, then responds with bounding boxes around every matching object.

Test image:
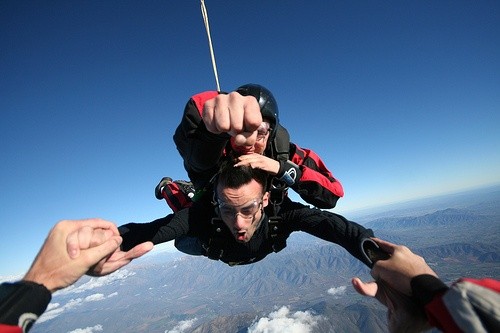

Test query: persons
[0,218,500,333]
[66,84,390,279]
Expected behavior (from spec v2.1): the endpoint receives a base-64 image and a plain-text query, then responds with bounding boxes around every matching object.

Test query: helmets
[236,83,278,150]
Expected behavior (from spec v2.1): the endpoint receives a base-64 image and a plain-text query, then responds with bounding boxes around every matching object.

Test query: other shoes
[155,177,173,200]
